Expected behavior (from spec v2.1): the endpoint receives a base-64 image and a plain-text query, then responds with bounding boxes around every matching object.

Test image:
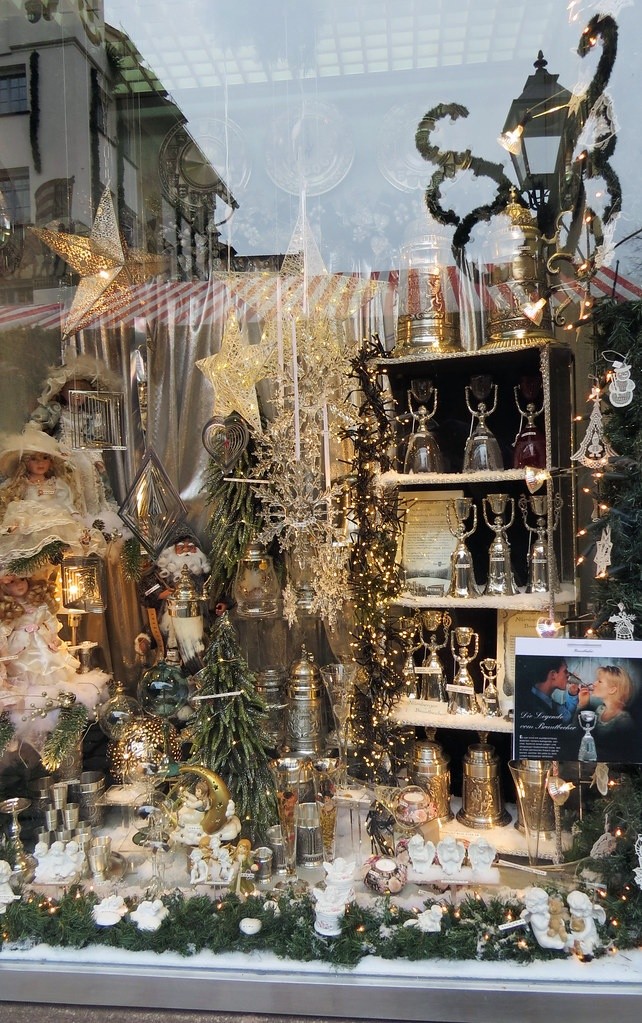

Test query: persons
[27,348,116,524]
[516,655,641,762]
[0,432,95,562]
[0,569,81,712]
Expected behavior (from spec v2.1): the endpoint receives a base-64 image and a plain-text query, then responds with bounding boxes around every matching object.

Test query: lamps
[106,715,183,787]
[50,557,104,649]
[206,191,398,384]
[195,312,279,441]
[26,179,189,349]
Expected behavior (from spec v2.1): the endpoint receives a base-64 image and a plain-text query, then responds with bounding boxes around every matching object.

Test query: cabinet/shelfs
[364,339,583,861]
